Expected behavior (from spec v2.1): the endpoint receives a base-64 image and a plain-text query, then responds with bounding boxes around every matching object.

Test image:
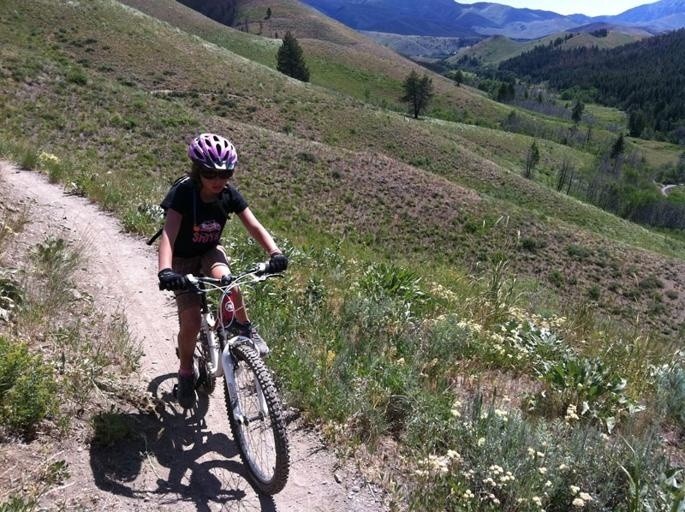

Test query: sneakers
[177,367,196,408]
[238,320,270,354]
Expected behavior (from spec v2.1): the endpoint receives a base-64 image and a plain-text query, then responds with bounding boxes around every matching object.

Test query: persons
[157,133,289,408]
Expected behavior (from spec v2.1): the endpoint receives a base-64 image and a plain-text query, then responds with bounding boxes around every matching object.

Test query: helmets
[187,132,238,172]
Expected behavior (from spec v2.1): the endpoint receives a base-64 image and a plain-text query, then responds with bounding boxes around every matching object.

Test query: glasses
[200,170,233,179]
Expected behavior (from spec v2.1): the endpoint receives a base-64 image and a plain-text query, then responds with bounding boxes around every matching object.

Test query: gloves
[158,267,186,291]
[269,251,288,271]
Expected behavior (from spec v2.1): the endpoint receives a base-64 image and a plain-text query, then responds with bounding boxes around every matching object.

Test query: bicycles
[155,261,293,498]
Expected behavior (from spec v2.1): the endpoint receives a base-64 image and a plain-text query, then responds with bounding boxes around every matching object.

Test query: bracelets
[268,249,279,255]
